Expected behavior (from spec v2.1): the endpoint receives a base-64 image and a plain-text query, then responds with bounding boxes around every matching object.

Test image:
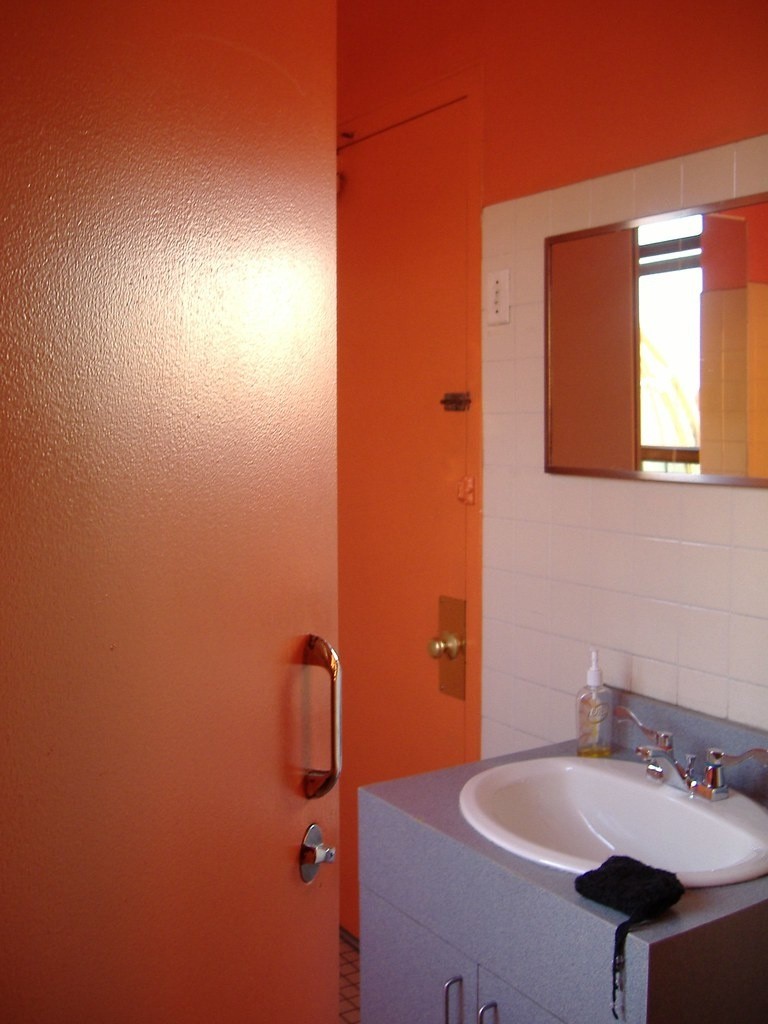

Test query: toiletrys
[574,645,614,758]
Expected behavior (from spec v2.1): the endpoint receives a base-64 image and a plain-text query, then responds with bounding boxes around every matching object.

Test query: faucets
[634,745,699,795]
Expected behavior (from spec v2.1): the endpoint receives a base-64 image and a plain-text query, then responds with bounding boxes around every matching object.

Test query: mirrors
[543,191,768,489]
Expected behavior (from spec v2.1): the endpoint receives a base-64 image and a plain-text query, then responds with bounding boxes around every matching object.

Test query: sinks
[459,756,768,888]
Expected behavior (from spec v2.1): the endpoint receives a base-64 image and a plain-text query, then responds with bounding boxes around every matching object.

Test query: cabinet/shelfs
[358,881,566,1024]
